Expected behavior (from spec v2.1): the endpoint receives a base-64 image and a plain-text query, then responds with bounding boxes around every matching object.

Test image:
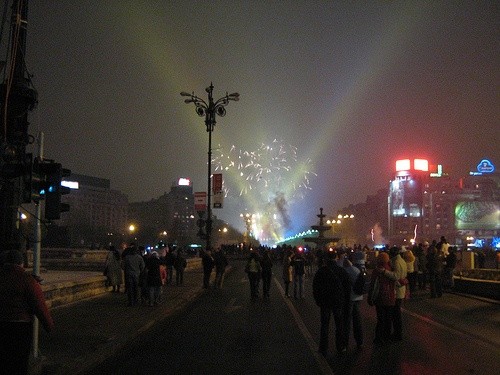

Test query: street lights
[179,82,240,263]
[326,219,342,247]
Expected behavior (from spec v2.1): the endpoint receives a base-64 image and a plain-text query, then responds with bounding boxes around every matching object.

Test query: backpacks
[354,267,372,295]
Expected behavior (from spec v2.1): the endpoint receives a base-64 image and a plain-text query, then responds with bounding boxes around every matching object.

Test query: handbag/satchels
[367,269,381,307]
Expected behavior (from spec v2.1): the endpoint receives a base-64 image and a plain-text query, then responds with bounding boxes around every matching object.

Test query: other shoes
[339,334,403,353]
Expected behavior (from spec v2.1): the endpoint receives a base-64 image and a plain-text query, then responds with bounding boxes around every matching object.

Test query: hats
[378,252,390,264]
[391,247,400,257]
[355,251,366,260]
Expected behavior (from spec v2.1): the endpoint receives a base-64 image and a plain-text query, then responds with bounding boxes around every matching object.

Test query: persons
[103,236,186,308]
[246,251,273,299]
[202,250,214,288]
[289,250,309,298]
[282,249,293,297]
[221,242,399,270]
[310,246,408,352]
[214,251,229,288]
[400,236,457,298]
[0,250,55,375]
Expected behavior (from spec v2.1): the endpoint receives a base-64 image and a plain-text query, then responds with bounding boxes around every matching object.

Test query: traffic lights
[19,152,44,203]
[45,162,70,220]
